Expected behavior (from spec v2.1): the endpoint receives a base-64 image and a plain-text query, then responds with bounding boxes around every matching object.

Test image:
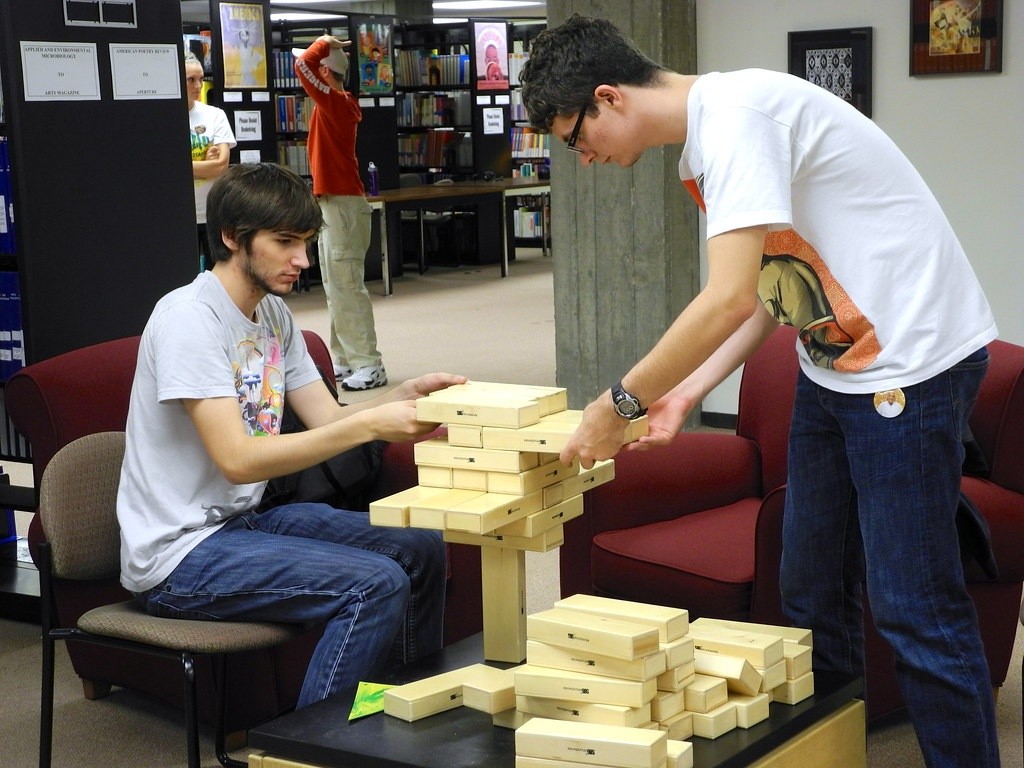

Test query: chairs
[39,431,301,768]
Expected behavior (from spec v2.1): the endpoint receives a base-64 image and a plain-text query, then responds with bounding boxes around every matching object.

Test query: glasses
[566,81,612,153]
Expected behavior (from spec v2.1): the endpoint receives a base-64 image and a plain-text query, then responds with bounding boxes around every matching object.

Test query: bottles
[368,161,378,196]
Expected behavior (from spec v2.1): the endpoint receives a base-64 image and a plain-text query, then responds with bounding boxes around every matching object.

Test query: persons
[183,52,238,269]
[877,391,902,418]
[114,165,467,708]
[292,35,387,390]
[477,45,508,80]
[520,16,1000,768]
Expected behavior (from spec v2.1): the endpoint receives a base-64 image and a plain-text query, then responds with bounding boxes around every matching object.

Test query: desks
[353,186,508,296]
[247,633,866,768]
[434,178,551,258]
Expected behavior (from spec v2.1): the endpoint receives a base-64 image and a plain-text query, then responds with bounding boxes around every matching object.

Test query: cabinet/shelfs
[181,0,549,295]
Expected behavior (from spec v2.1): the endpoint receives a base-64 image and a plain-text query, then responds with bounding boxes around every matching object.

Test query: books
[0,141,33,458]
[272,48,317,192]
[509,51,550,239]
[394,47,473,168]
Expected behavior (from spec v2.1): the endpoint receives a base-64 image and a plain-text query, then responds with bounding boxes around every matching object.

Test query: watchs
[611,377,648,420]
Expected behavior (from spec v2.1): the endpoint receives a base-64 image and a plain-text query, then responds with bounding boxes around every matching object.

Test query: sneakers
[333,365,354,381]
[341,362,388,391]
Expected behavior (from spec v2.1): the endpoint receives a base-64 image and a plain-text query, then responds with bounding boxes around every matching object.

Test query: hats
[292,34,348,75]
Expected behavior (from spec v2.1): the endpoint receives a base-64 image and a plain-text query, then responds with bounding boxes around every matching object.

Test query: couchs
[4,315,1023,736]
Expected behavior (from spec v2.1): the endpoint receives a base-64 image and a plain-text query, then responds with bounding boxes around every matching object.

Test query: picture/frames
[787,26,872,121]
[909,0,1001,77]
